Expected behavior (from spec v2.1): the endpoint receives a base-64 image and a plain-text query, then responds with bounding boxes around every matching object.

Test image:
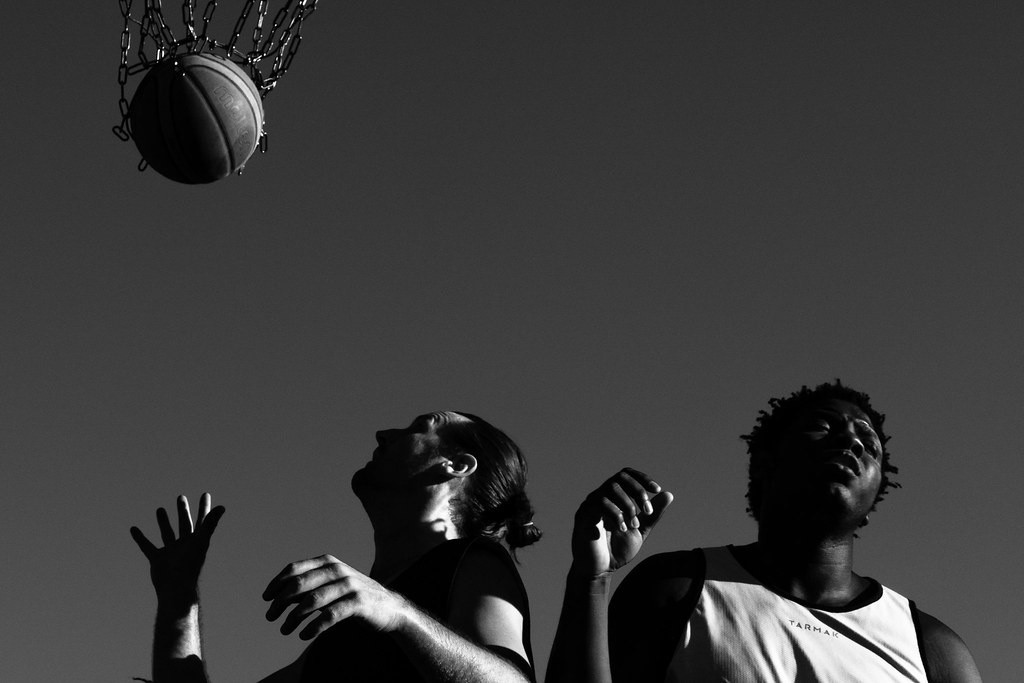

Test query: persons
[545,382,981,683]
[130,410,542,683]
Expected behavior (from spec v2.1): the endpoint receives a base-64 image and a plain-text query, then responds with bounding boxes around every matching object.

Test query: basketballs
[126,52,267,188]
